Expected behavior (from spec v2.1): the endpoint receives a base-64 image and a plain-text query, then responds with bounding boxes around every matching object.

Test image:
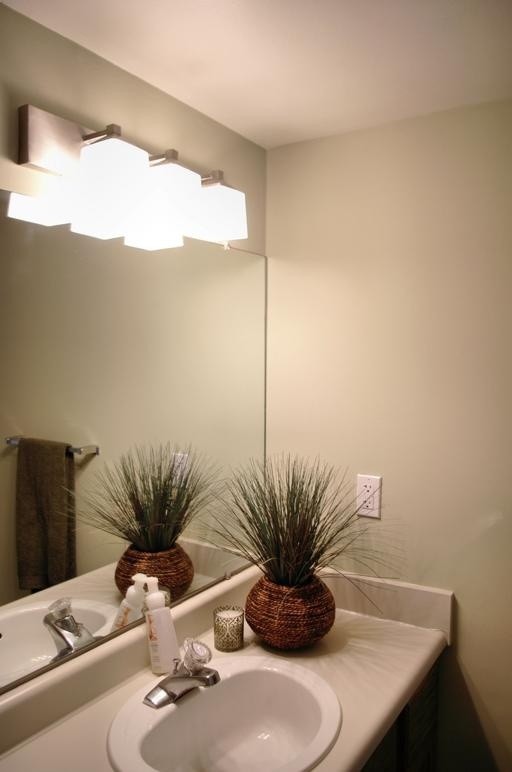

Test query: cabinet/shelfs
[358,646,441,772]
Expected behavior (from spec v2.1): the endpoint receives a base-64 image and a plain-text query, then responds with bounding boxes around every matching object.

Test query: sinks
[105,655,344,772]
[0,601,119,688]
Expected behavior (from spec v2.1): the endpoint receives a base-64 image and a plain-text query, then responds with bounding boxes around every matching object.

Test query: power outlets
[354,472,384,522]
[166,449,192,488]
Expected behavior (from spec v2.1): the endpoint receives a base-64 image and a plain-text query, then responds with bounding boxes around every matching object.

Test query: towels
[14,435,78,595]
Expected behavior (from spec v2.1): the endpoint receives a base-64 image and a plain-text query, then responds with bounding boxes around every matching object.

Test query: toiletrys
[144,577,182,673]
[109,573,147,633]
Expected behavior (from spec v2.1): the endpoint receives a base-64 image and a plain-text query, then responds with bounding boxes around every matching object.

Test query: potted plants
[52,436,226,604]
[192,449,406,652]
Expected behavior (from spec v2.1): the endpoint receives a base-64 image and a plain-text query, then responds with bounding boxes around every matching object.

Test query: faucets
[142,639,220,709]
[42,595,104,662]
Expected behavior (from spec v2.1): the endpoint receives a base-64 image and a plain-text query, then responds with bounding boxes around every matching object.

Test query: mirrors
[0,185,266,697]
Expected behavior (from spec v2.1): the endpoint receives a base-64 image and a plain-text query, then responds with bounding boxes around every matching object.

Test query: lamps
[18,102,248,251]
